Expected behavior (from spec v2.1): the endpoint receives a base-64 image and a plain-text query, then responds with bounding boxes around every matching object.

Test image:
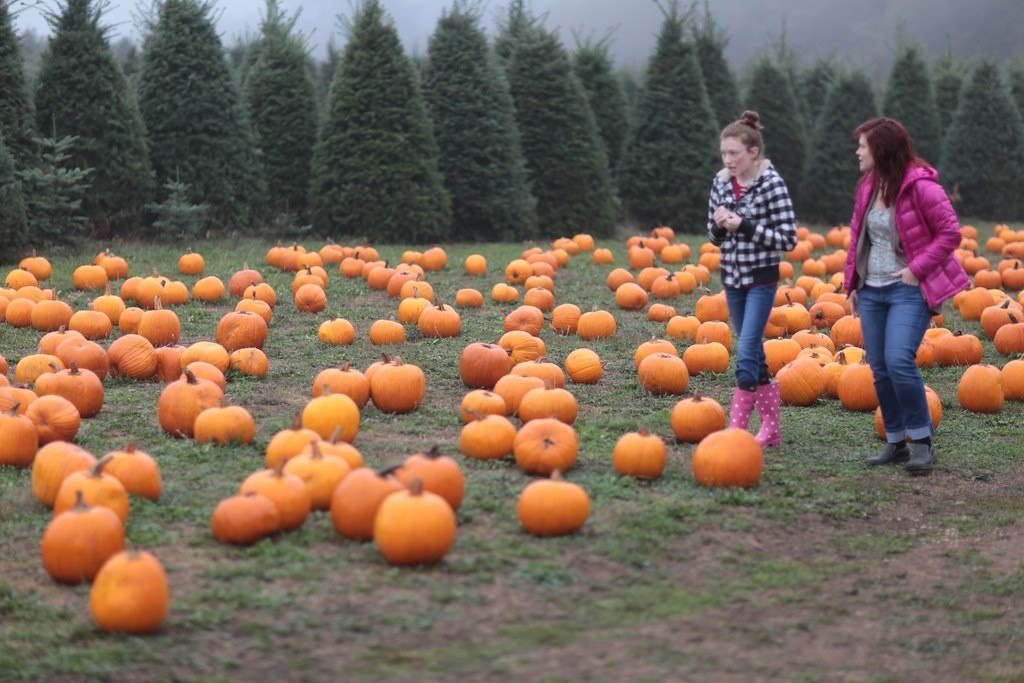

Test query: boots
[727,380,783,450]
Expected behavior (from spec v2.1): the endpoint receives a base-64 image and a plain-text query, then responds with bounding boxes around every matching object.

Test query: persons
[706,111,798,448]
[843,118,970,475]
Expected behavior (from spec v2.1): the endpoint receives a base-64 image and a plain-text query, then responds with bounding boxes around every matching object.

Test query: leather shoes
[906,442,938,476]
[864,442,911,466]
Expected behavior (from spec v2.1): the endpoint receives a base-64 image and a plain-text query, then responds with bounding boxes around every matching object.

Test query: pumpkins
[0,223,764,634]
[763,221,887,442]
[914,224,1024,433]
